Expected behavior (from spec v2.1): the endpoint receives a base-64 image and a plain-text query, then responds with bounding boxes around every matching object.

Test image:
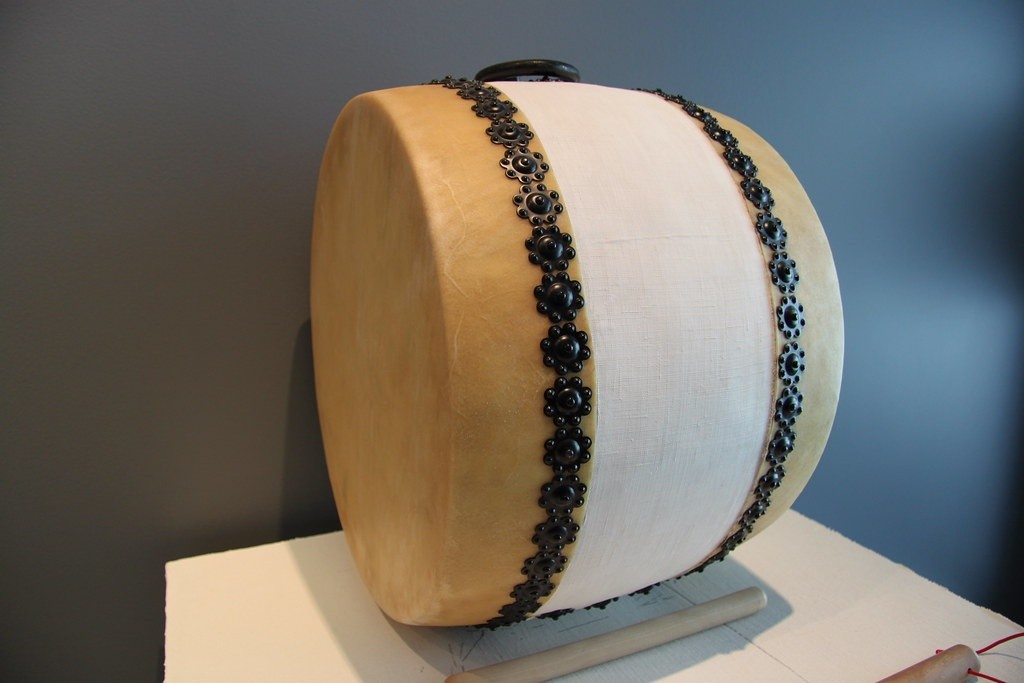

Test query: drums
[305,74,851,634]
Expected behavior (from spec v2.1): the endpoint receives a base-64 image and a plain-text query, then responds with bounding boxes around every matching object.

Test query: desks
[166,508,1024,683]
